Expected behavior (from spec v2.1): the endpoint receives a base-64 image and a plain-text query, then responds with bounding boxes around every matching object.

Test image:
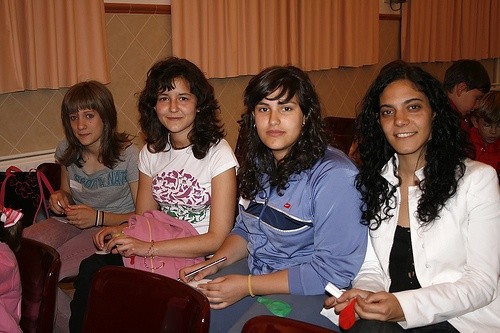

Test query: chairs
[0,116,361,333]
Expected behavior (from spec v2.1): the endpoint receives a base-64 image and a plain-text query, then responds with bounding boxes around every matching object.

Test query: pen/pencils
[177,257,228,281]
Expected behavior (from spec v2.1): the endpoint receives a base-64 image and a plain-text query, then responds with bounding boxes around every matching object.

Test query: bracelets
[95,210,104,226]
[247,273,255,297]
[144,240,165,256]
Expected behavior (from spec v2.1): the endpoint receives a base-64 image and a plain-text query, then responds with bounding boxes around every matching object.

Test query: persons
[178,65,368,333]
[441,59,500,184]
[68,56,240,333]
[322,67,500,333]
[21,80,140,284]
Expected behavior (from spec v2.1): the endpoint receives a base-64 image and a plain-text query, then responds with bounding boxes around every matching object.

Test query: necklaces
[168,133,192,150]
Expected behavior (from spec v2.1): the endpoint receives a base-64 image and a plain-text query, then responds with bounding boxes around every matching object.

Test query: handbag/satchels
[0,166,55,224]
